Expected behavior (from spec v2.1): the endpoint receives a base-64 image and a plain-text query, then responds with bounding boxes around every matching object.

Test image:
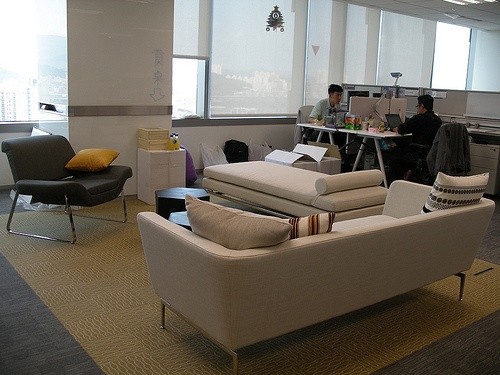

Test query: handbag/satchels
[224,140,248,163]
[248,136,273,161]
[201,143,229,169]
[180,144,198,186]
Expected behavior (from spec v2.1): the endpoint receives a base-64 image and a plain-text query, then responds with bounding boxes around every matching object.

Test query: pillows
[65,148,120,172]
[420,171,489,214]
[282,212,335,240]
[184,193,292,251]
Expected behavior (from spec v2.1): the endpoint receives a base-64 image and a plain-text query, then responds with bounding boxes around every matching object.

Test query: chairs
[293,106,314,146]
[1,135,133,244]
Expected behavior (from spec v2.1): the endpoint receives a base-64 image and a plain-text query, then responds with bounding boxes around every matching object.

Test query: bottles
[169,131,179,150]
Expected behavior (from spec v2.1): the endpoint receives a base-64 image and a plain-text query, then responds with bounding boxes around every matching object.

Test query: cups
[345,115,361,130]
[362,122,369,131]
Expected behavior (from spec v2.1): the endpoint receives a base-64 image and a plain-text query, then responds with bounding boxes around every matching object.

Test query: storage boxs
[138,128,170,150]
[265,141,342,175]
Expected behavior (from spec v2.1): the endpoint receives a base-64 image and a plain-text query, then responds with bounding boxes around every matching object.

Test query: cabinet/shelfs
[472,143,500,195]
[137,148,186,206]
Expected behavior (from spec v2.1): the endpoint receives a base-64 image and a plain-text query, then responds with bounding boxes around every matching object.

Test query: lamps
[373,72,402,124]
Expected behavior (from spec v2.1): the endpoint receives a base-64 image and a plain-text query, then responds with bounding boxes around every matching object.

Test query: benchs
[136,162,495,375]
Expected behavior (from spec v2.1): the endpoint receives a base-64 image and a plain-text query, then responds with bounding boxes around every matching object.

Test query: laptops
[385,114,402,132]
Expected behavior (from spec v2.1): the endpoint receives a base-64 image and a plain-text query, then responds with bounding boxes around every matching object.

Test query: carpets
[0,197,500,375]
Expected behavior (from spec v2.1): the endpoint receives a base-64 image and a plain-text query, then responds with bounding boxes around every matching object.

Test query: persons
[385,94,442,182]
[306,84,349,172]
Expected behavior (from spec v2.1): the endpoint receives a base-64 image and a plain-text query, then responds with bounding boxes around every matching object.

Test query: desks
[297,123,412,189]
[467,127,500,136]
[168,211,192,231]
[155,187,210,220]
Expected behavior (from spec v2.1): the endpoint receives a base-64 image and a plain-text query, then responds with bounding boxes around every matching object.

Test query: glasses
[416,104,421,108]
